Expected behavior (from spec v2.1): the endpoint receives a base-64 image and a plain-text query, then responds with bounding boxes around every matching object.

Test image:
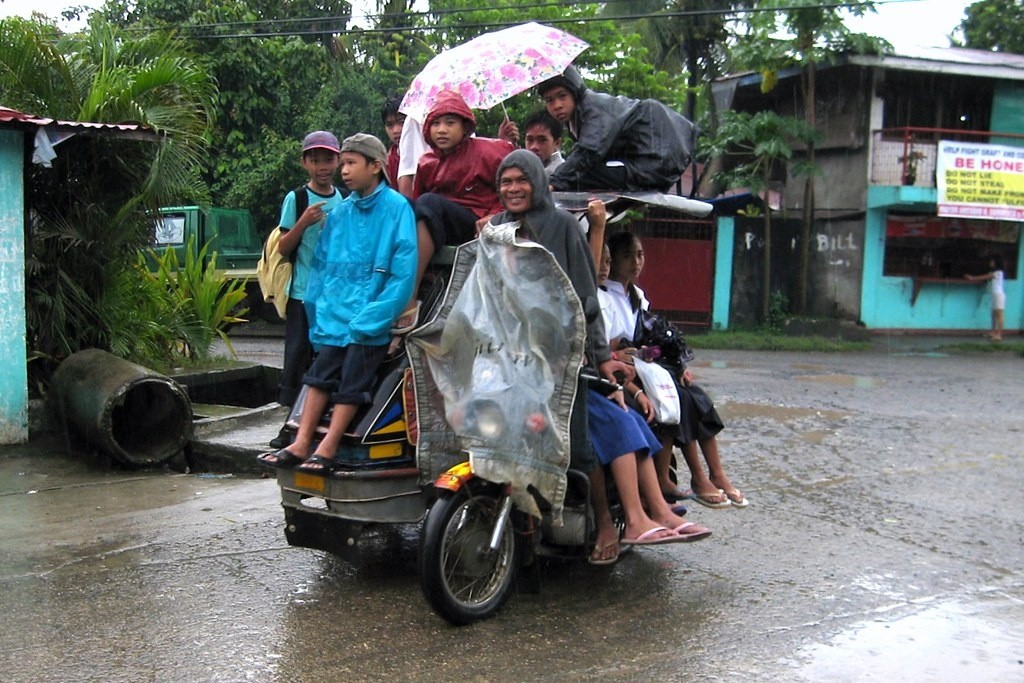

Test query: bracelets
[633,390,643,400]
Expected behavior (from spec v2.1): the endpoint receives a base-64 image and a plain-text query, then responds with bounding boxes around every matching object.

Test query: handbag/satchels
[632,355,681,425]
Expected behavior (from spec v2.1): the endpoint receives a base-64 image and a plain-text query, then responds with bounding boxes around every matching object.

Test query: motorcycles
[275,192,677,628]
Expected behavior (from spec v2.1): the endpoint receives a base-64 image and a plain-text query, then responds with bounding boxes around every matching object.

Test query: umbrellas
[396,22,590,148]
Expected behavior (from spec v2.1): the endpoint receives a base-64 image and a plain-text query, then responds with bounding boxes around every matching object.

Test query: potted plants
[896,134,928,185]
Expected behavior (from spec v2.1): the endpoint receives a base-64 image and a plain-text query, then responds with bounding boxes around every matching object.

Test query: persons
[255,66,751,565]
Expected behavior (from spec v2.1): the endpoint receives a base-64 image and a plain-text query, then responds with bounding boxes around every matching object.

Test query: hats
[303,130,341,154]
[341,132,391,185]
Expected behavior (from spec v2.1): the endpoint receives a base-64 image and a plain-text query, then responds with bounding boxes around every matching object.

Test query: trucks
[120,206,263,336]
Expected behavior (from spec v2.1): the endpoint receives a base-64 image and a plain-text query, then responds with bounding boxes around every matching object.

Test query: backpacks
[256,185,350,320]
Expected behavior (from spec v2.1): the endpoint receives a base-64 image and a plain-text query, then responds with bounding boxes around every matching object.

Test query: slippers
[688,488,731,509]
[620,526,687,545]
[256,448,306,468]
[388,300,422,333]
[588,528,621,564]
[661,486,694,500]
[296,454,339,473]
[666,504,687,517]
[725,488,750,507]
[672,522,712,542]
